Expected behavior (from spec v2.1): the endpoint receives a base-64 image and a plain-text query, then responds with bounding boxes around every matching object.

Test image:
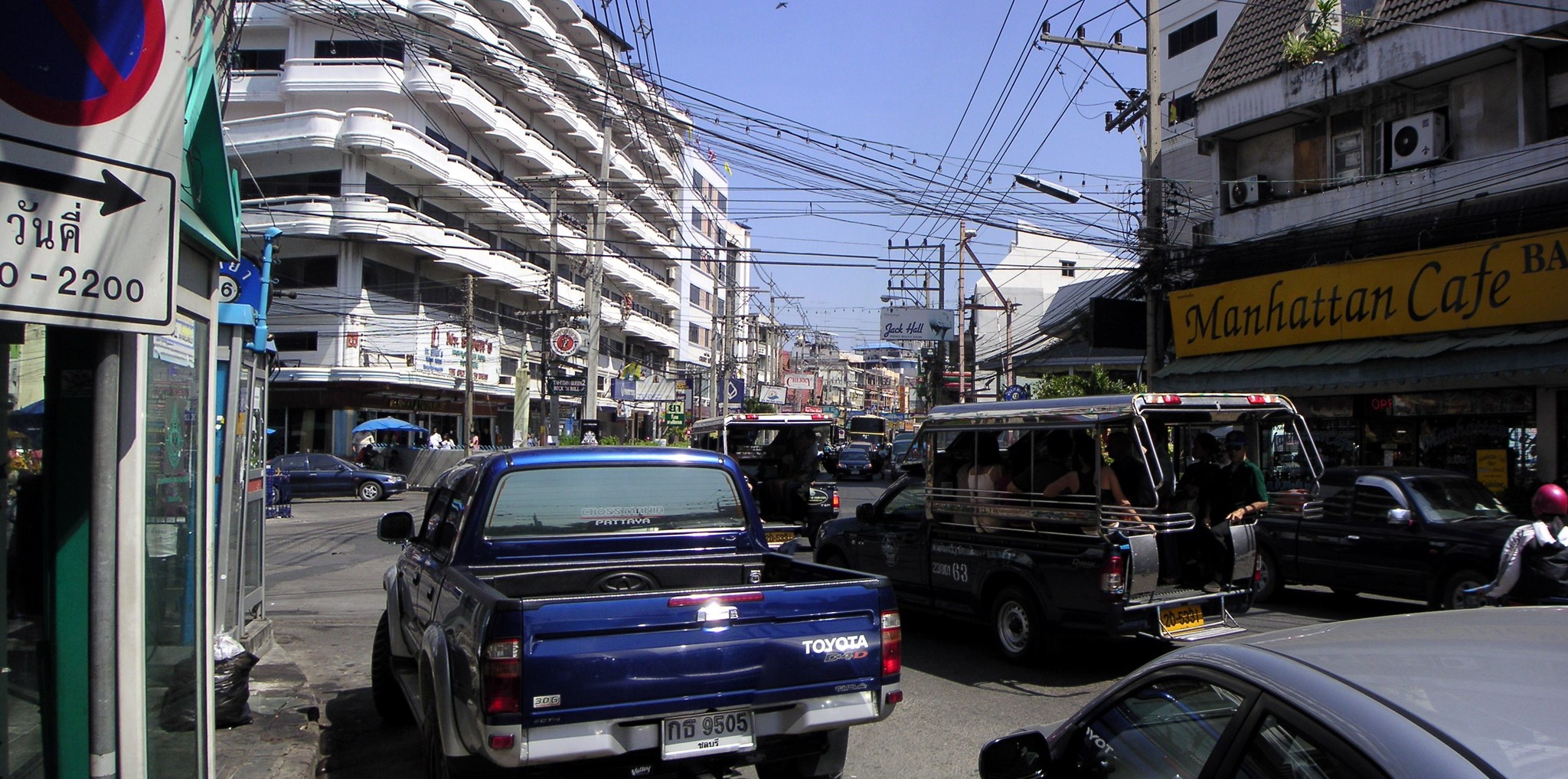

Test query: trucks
[814,393,1328,665]
[690,412,840,549]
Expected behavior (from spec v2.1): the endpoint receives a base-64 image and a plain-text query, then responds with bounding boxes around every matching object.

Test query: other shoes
[760,519,766,525]
[1163,569,1187,584]
[1204,572,1241,591]
[794,520,802,525]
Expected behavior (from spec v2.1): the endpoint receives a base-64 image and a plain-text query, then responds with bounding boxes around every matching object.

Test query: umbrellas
[354,415,428,434]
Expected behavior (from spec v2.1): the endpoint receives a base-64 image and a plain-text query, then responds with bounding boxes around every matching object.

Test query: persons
[351,428,490,472]
[1465,484,1568,607]
[947,430,1269,594]
[527,433,535,446]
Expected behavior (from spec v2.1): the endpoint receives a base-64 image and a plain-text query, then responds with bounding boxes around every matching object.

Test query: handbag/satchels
[1169,498,1199,515]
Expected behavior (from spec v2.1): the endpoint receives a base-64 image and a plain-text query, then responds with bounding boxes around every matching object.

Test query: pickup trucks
[372,448,904,779]
[1259,466,1535,611]
[267,451,409,506]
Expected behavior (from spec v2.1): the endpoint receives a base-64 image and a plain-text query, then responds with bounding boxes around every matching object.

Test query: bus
[848,414,889,446]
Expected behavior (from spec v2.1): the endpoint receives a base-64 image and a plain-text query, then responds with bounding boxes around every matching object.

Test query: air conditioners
[1392,112,1448,170]
[1228,175,1272,207]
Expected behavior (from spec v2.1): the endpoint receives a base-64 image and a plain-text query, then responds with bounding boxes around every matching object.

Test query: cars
[978,603,1568,779]
[822,441,884,483]
[891,433,926,481]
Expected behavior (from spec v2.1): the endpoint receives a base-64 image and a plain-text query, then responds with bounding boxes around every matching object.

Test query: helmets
[1532,484,1568,518]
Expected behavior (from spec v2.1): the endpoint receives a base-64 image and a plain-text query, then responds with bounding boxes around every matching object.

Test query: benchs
[931,500,1126,557]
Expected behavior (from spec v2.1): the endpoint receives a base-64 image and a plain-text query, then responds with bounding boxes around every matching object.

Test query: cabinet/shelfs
[1332,128,1363,185]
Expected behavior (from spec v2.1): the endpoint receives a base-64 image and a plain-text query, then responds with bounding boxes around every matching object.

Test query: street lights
[1012,173,1164,392]
[879,295,934,411]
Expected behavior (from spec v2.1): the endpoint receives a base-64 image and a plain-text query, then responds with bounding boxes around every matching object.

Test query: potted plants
[1287,0,1373,67]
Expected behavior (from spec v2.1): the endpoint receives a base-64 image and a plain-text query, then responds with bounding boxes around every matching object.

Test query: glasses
[1230,445,1245,451]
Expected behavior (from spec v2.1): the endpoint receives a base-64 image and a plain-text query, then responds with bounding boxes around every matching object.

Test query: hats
[1226,430,1248,446]
